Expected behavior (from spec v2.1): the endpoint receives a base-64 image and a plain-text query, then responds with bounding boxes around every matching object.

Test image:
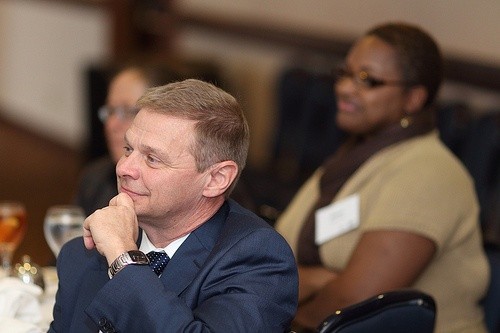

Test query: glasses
[333,66,412,91]
[98,105,140,124]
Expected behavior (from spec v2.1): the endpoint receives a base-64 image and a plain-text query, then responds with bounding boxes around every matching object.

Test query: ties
[143,250,170,276]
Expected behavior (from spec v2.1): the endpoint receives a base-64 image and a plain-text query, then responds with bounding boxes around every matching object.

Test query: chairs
[316,290,436,333]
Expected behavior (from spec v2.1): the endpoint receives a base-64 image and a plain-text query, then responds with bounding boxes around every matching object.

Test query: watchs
[107,250,150,279]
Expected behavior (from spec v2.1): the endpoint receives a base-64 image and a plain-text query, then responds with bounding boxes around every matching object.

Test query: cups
[43,204,86,261]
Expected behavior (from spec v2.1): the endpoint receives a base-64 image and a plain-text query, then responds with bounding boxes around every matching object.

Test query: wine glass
[0,200,29,277]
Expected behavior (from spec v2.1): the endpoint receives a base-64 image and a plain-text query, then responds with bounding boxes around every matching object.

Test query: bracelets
[287,325,296,333]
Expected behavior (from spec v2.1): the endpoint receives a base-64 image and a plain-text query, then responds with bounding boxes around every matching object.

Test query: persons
[46,79,299,333]
[48,59,182,266]
[275,22,490,333]
